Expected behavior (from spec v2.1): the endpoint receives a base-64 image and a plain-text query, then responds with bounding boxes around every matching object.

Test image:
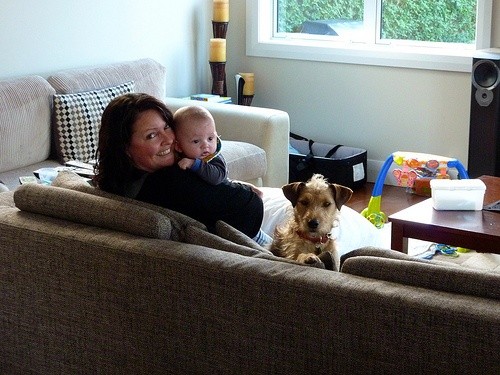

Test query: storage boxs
[430,178,487,210]
[289,139,368,193]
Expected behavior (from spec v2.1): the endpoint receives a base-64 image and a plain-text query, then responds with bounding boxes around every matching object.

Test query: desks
[388,175,500,255]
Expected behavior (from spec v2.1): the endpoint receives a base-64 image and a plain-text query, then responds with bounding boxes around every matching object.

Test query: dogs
[266,173,355,268]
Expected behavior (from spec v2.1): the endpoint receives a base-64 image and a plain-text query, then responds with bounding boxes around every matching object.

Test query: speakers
[467,48,500,180]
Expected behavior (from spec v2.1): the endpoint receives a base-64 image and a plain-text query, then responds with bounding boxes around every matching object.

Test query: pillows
[52,80,140,164]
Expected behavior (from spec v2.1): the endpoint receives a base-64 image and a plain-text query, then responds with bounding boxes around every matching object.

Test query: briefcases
[289,132,368,192]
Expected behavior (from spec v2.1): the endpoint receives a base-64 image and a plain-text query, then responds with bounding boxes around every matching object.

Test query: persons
[173,106,228,184]
[92,93,264,240]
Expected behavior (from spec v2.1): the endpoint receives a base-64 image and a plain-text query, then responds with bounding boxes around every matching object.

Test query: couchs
[0,57,500,375]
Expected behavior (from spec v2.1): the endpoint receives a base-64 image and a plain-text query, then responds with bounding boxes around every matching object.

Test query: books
[190,94,220,102]
